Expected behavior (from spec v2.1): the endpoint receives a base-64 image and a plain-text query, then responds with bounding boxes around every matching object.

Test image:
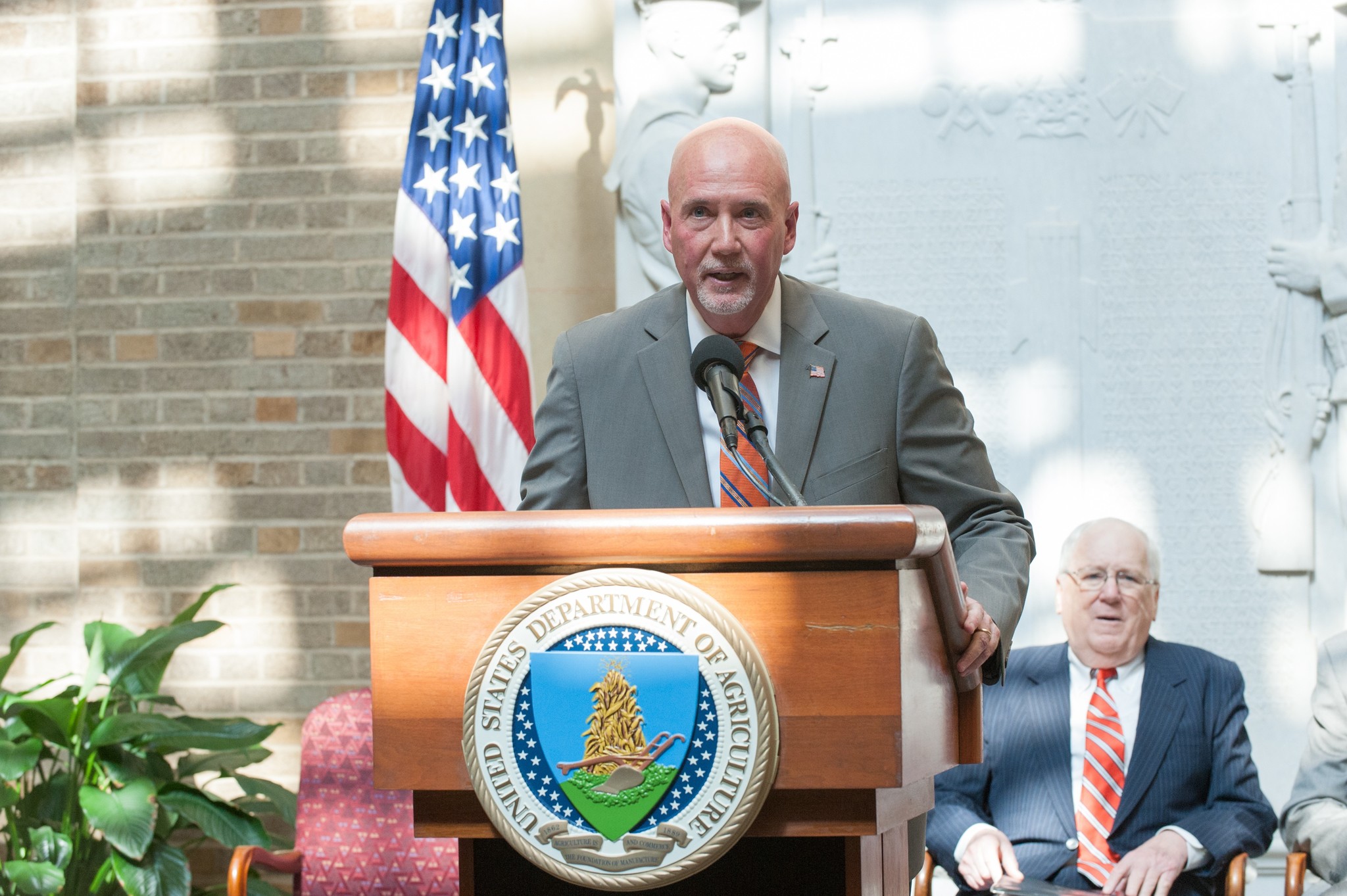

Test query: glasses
[1064,568,1159,596]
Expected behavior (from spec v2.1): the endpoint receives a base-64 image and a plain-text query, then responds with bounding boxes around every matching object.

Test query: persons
[924,517,1278,896]
[1258,0,1346,658]
[515,118,1037,689]
[602,0,844,313]
[1278,633,1347,896]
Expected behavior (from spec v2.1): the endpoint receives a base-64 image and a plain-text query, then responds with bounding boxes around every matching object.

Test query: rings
[974,628,992,639]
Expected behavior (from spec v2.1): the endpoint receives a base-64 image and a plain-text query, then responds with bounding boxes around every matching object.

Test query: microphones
[688,334,745,451]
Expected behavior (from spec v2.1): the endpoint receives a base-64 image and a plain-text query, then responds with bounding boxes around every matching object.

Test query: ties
[1077,667,1126,887]
[720,341,772,507]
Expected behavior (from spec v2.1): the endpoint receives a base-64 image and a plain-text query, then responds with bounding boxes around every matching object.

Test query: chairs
[226,685,459,896]
[912,844,1249,896]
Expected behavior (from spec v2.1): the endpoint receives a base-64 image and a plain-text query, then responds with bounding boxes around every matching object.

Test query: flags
[384,0,536,513]
